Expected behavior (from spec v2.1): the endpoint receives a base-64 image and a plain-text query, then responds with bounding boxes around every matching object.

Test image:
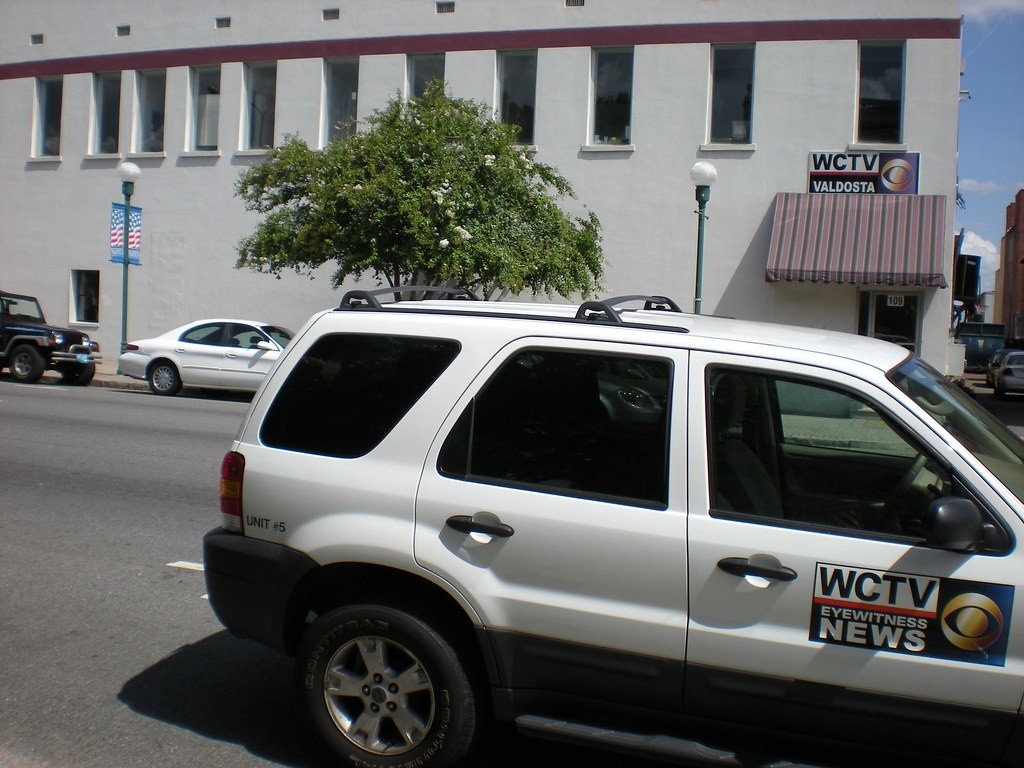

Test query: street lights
[684,159,721,312]
[116,160,142,375]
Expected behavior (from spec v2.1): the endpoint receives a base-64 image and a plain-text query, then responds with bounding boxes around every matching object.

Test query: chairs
[710,374,784,521]
[745,379,860,506]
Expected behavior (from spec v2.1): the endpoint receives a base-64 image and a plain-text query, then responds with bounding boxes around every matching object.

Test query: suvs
[201,288,1024,768]
[0,290,104,383]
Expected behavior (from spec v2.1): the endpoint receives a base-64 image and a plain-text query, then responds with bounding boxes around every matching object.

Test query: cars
[988,345,1024,398]
[117,319,301,395]
